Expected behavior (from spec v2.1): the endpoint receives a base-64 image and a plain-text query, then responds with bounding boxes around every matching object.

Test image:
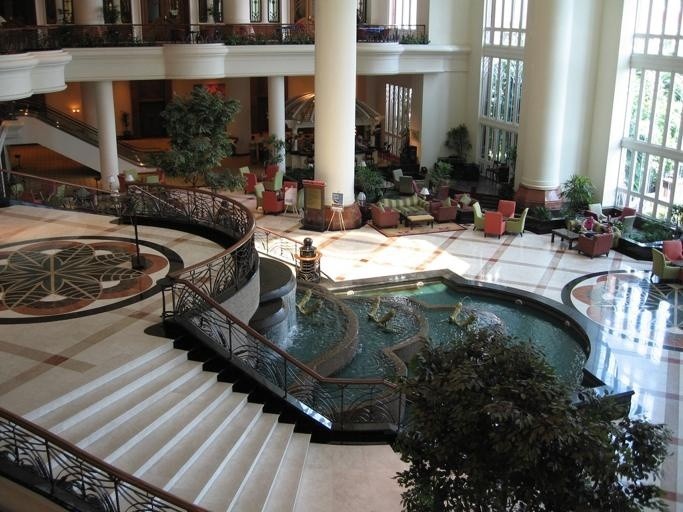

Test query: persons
[606,221,614,233]
[570,227,604,250]
[256,132,265,162]
[584,216,594,231]
[247,135,257,165]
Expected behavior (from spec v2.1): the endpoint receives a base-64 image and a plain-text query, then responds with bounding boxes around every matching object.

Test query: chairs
[238,155,529,240]
[576,203,683,286]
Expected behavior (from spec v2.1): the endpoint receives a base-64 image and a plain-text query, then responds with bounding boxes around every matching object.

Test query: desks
[551,228,579,250]
